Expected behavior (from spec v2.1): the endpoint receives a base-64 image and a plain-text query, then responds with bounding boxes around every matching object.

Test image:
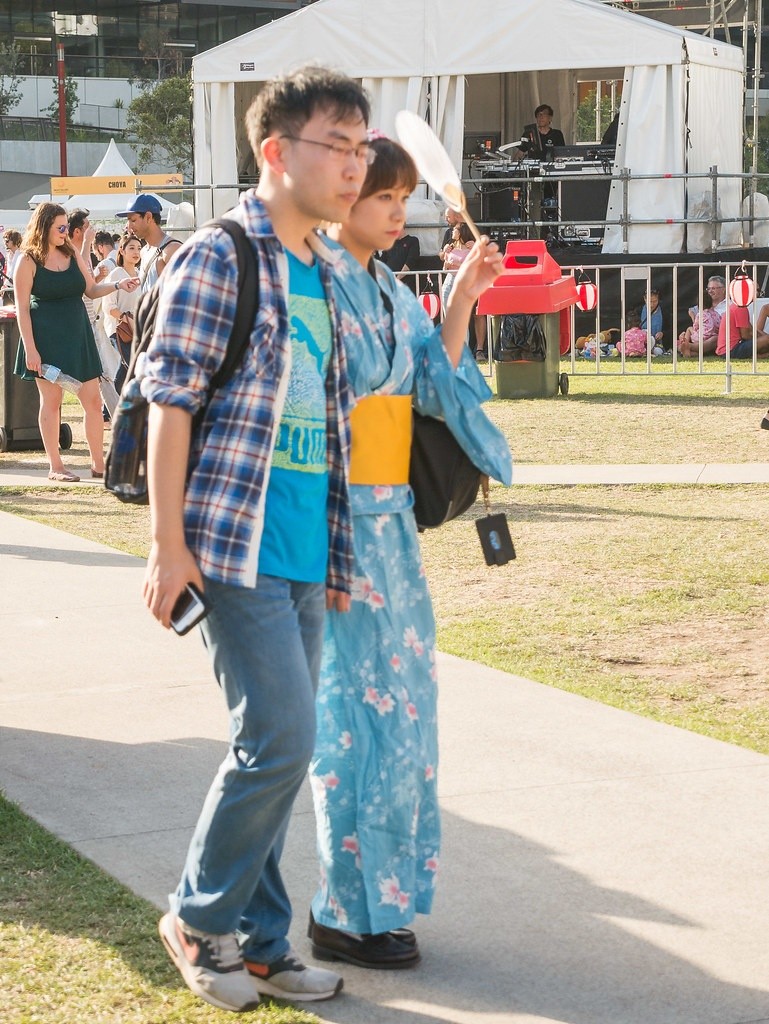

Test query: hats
[115,194,162,217]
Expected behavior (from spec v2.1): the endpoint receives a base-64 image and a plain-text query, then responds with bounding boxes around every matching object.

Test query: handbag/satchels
[116,311,134,343]
[409,408,480,533]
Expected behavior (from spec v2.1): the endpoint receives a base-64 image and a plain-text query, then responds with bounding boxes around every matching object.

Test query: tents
[60,138,178,221]
[192,0,745,253]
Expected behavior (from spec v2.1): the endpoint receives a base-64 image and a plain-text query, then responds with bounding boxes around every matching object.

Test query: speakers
[480,169,544,242]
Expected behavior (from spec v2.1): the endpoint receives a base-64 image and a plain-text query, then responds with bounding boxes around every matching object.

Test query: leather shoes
[308,910,422,970]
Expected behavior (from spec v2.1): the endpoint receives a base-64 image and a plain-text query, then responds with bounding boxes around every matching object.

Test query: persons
[0,195,184,482]
[138,66,513,1012]
[380,222,420,296]
[616,287,670,357]
[677,276,769,358]
[439,207,486,360]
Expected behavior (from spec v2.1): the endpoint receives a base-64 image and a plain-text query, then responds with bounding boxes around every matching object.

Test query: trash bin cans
[476,241,581,399]
[0,305,72,452]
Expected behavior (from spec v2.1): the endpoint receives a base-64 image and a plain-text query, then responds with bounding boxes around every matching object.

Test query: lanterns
[418,291,439,320]
[575,281,598,312]
[730,276,753,308]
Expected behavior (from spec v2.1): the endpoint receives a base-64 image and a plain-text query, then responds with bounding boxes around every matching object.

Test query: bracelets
[115,281,119,290]
[118,311,125,323]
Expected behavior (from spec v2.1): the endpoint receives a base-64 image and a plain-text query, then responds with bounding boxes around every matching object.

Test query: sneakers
[158,911,260,1012]
[245,955,344,1001]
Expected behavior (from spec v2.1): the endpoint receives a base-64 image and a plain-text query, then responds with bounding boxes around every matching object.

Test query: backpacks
[104,218,260,505]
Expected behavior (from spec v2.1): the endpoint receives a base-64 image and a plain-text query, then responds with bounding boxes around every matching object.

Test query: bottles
[41,364,83,395]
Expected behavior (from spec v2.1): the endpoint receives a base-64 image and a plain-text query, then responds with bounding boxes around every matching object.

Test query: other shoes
[48,471,80,482]
[91,469,103,478]
[104,421,112,430]
[476,350,485,360]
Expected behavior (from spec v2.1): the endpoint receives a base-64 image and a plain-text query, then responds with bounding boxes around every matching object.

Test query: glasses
[52,223,70,233]
[706,286,723,291]
[536,112,552,117]
[279,134,377,165]
[3,239,11,243]
[644,290,657,294]
[453,227,459,230]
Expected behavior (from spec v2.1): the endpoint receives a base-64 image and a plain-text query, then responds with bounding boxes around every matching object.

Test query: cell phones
[168,582,213,636]
